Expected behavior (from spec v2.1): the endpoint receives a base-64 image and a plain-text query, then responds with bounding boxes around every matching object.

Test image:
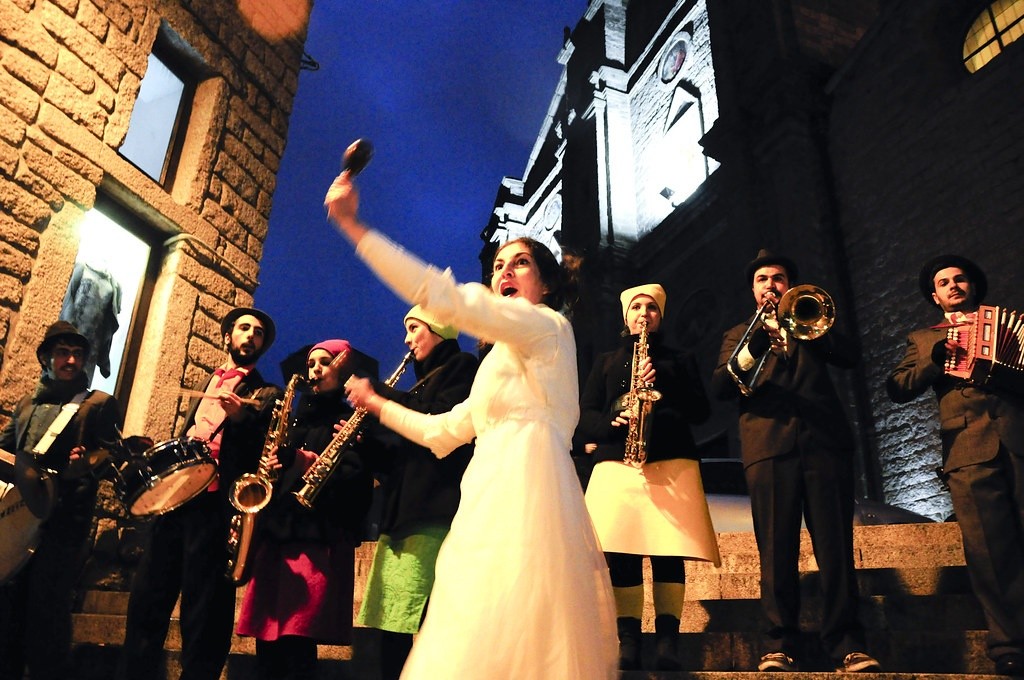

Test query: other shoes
[758,651,799,672]
[843,651,882,673]
[995,653,1024,677]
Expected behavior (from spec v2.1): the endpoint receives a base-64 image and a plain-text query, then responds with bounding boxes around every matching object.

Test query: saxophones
[224,374,321,584]
[625,318,650,469]
[291,348,416,510]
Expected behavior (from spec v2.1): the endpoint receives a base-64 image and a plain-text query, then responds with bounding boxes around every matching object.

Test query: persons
[571,281,720,671]
[715,247,884,673]
[322,139,620,680]
[116,305,281,680]
[329,302,479,680]
[240,337,371,680]
[887,253,1024,676]
[0,321,119,680]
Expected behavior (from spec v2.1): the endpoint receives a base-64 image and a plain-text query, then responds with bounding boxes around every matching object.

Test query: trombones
[727,285,838,399]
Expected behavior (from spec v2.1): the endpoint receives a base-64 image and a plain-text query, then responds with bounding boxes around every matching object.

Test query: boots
[617,617,644,672]
[655,614,682,670]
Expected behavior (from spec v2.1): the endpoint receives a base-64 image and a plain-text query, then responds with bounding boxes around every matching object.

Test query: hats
[746,248,799,289]
[919,255,988,307]
[36,321,90,373]
[404,304,460,341]
[220,307,276,359]
[307,338,351,371]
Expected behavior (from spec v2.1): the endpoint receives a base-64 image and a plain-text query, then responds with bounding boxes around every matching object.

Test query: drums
[112,435,219,522]
[0,444,59,587]
